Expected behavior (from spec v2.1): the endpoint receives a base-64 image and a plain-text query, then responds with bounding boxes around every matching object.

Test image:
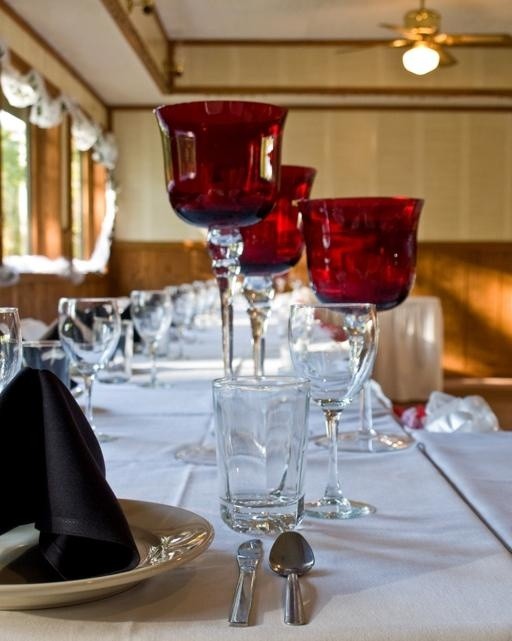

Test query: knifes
[230,539,264,628]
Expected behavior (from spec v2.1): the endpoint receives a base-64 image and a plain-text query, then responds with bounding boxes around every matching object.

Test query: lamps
[397,41,445,78]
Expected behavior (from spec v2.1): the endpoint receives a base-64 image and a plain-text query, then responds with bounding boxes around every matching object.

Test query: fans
[332,0,511,66]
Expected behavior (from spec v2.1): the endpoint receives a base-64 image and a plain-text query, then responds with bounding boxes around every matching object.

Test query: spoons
[267,532,315,626]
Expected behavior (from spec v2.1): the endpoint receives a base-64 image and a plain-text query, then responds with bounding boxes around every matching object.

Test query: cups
[154,100,290,466]
[287,303,378,520]
[91,297,134,387]
[1,307,24,394]
[131,290,174,390]
[291,196,427,455]
[238,165,319,378]
[368,297,445,433]
[163,285,197,364]
[58,298,122,444]
[211,376,311,537]
[22,341,73,394]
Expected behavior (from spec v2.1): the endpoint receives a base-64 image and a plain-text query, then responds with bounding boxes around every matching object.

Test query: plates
[0,499,215,613]
